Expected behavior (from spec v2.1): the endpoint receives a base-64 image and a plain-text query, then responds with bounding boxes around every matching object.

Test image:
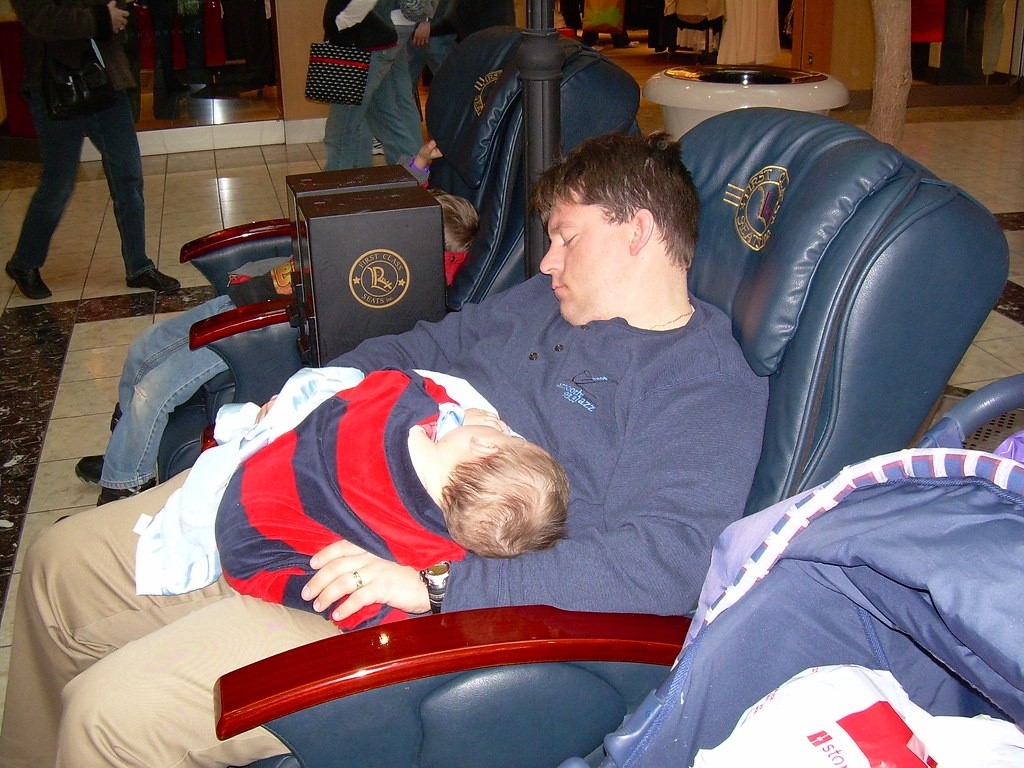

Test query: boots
[97,478,156,507]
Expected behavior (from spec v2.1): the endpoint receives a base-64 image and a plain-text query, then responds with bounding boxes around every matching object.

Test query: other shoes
[624,41,639,48]
[591,45,604,51]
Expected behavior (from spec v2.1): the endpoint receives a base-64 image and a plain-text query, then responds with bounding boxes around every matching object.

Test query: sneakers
[373,137,384,154]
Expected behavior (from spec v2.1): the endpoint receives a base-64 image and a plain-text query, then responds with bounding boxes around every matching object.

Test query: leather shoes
[127,269,180,292]
[6,260,52,298]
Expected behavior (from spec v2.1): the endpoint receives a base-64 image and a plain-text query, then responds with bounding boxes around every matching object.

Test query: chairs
[157,27,1024,768]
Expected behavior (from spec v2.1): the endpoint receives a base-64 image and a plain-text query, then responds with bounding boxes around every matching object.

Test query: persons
[55,140,480,524]
[213,367,571,635]
[4,0,182,299]
[0,127,770,768]
[318,0,516,172]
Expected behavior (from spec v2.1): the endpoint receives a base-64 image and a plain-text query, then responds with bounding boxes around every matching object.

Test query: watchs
[419,557,455,617]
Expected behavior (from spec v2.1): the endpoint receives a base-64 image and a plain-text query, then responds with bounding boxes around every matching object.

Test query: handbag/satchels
[74,402,122,483]
[304,43,370,105]
